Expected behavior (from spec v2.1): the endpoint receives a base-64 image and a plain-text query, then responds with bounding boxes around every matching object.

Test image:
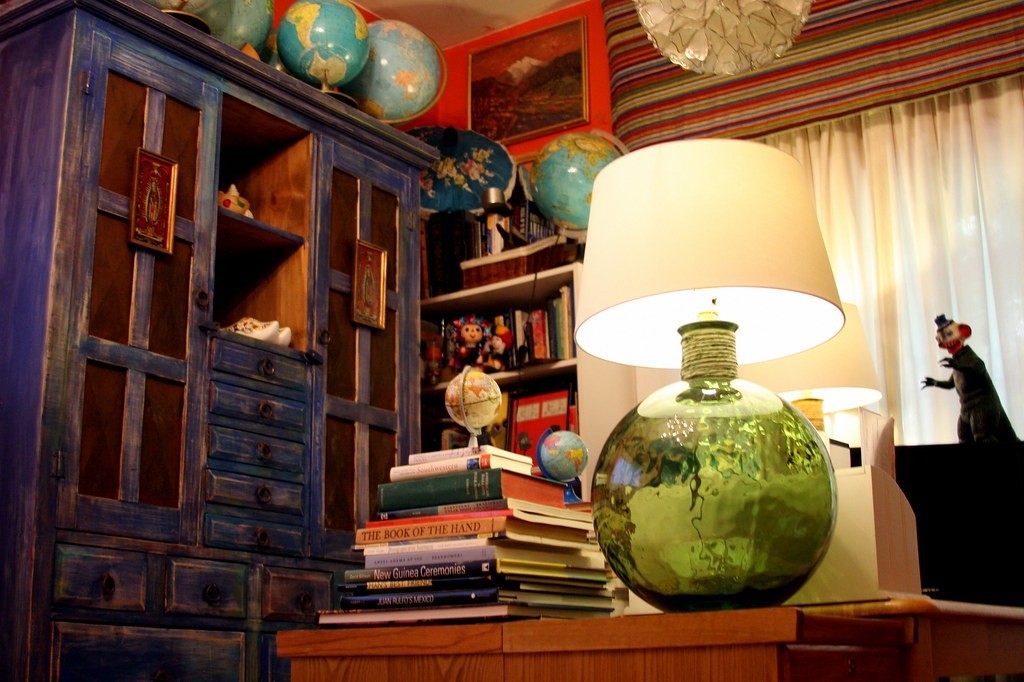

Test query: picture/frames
[129,146,179,257]
[348,237,389,331]
[466,16,589,146]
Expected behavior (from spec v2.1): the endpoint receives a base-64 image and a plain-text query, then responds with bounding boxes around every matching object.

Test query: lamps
[572,137,847,612]
[632,0,814,77]
[735,299,883,449]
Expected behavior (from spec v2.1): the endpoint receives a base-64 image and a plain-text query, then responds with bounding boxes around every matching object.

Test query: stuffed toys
[449,316,503,376]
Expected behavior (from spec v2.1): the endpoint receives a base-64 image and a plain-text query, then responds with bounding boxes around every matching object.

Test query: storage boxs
[628,465,924,616]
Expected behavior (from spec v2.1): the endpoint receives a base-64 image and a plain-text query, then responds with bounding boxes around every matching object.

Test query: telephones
[444,364,503,449]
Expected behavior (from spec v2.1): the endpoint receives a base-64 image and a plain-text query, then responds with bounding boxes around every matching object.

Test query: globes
[276,0,373,108]
[528,127,632,238]
[536,428,589,501]
[338,18,450,125]
[145,0,274,53]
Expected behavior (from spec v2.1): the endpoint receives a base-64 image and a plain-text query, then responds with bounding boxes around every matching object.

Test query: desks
[276,597,1024,682]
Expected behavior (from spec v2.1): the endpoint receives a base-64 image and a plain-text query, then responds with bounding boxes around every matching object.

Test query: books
[425,390,579,477]
[495,283,572,370]
[318,444,615,624]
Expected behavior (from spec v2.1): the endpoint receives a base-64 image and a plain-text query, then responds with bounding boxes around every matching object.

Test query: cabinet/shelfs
[0,0,437,682]
[419,262,639,505]
